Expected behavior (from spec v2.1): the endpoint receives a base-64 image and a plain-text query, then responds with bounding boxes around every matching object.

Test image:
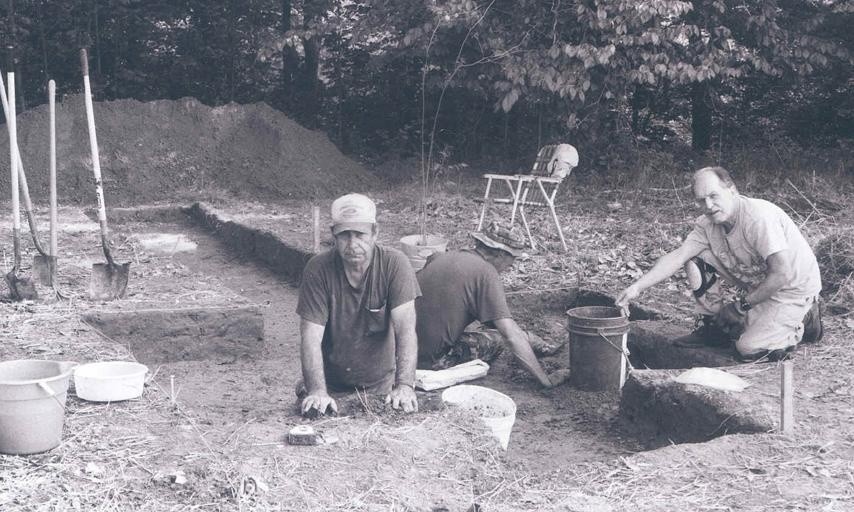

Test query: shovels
[0,59,56,299]
[80,47,131,300]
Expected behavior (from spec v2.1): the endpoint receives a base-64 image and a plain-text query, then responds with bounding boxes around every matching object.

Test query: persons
[293,191,426,418]
[613,166,825,364]
[412,216,569,388]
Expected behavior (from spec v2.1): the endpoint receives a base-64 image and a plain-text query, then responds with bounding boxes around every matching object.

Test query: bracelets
[739,298,754,310]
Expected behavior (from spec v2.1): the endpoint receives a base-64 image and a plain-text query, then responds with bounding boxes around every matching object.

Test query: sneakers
[802,303,823,342]
[674,321,727,348]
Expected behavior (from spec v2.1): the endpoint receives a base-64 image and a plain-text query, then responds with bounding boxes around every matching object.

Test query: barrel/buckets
[566,306,629,391]
[1,360,77,454]
[400,235,449,273]
[442,384,518,452]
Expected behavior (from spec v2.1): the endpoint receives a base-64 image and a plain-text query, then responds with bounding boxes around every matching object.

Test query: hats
[330,194,377,234]
[471,219,526,258]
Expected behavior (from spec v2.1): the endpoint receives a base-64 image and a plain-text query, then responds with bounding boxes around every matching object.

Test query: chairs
[475,143,578,255]
